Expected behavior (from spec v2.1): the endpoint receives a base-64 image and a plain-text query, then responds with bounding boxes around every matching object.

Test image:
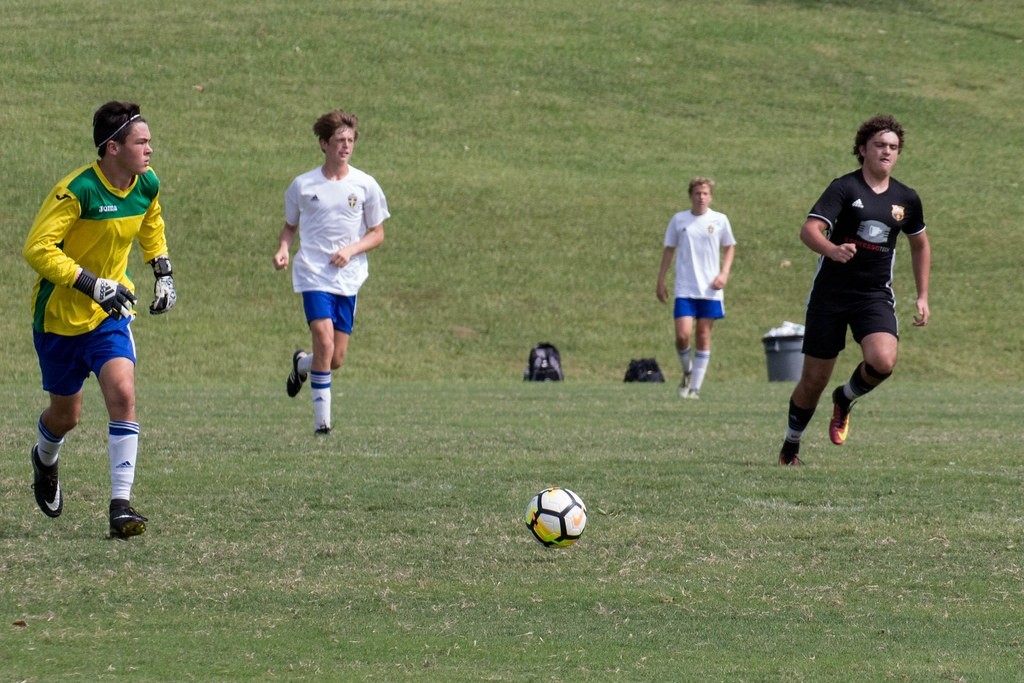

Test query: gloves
[71,269,138,321]
[149,257,177,316]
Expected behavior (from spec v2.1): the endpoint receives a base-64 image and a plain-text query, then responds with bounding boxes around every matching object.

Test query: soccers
[526,486,587,548]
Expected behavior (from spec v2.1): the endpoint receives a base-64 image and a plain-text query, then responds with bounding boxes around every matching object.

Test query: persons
[23,102,176,539]
[657,178,738,402]
[271,110,391,435]
[778,114,931,466]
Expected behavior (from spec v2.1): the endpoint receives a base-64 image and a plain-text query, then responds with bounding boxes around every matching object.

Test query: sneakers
[828,385,857,445]
[777,438,806,468]
[30,442,63,518]
[687,388,701,400]
[677,369,693,399]
[110,507,148,538]
[315,424,332,436]
[286,349,307,398]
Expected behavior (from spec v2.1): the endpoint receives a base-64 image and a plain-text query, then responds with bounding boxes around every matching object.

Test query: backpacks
[522,342,563,381]
[625,358,665,382]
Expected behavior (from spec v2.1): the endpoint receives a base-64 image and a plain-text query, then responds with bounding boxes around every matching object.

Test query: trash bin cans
[760,331,807,385]
